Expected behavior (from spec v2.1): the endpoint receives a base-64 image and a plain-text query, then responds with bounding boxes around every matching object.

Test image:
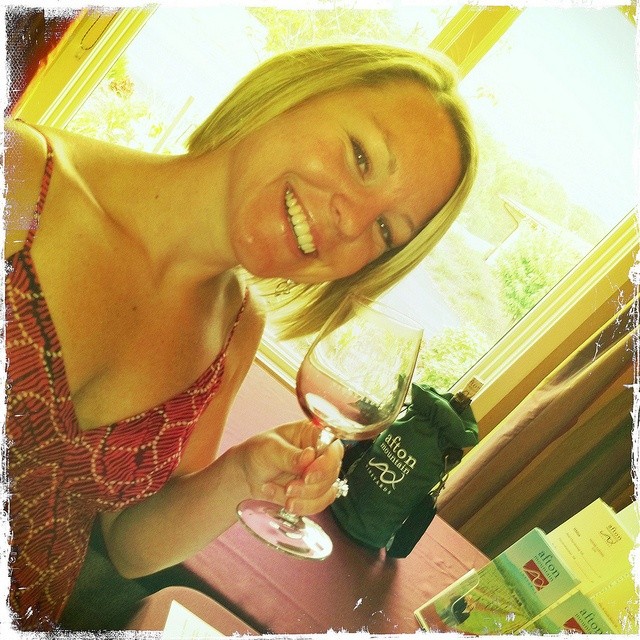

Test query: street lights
[332,382,478,557]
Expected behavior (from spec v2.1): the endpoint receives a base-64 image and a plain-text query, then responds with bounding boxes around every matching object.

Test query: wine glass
[331,478,349,498]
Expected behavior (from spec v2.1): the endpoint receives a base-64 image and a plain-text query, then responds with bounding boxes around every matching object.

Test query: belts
[236,294,425,563]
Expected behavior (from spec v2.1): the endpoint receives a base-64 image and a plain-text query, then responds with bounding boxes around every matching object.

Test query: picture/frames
[174,364,492,632]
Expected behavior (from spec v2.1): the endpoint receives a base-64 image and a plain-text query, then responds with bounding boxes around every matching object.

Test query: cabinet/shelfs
[448,379,484,414]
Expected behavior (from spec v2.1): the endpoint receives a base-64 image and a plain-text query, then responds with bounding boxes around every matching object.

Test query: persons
[6,40,483,629]
